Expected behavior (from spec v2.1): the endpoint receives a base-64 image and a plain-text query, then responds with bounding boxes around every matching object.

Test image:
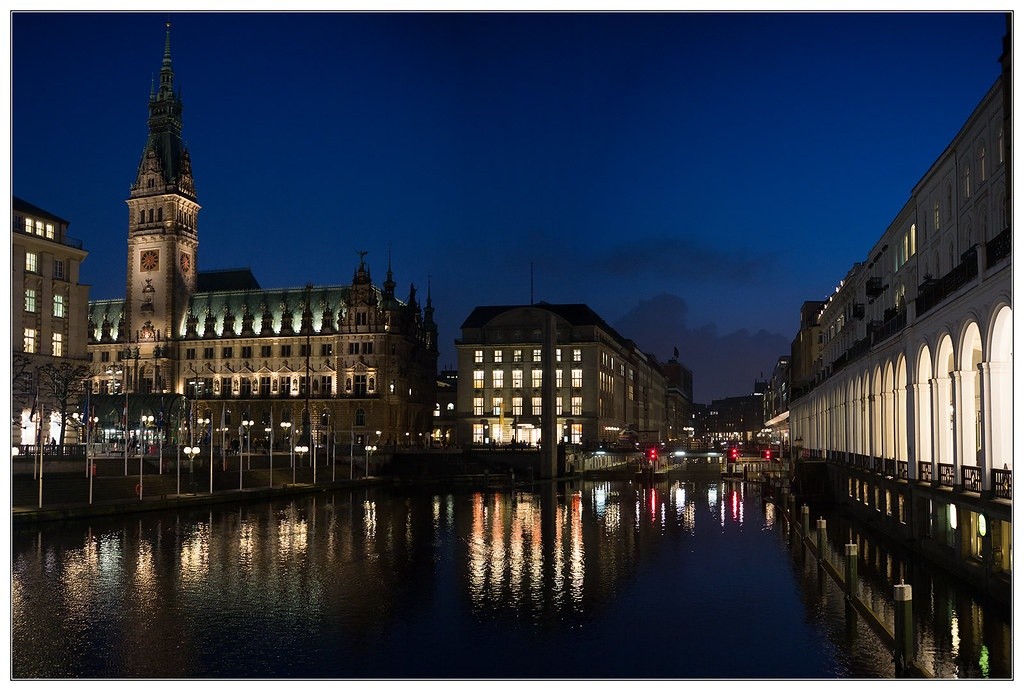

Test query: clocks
[140,249,159,272]
[178,248,192,275]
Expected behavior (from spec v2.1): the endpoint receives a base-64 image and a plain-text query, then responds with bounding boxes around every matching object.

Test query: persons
[145,444,151,455]
[51,438,56,454]
[165,436,174,453]
[252,438,270,454]
[276,440,279,450]
[225,438,240,455]
[111,435,137,458]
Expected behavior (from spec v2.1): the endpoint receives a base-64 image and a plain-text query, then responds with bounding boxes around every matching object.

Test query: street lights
[142,414,154,444]
[184,446,201,482]
[242,420,255,471]
[280,422,292,452]
[73,413,85,443]
[294,446,309,468]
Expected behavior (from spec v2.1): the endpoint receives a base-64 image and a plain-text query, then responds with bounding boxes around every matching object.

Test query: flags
[82,398,88,423]
[205,425,212,443]
[122,401,126,427]
[158,408,164,432]
[29,392,37,421]
